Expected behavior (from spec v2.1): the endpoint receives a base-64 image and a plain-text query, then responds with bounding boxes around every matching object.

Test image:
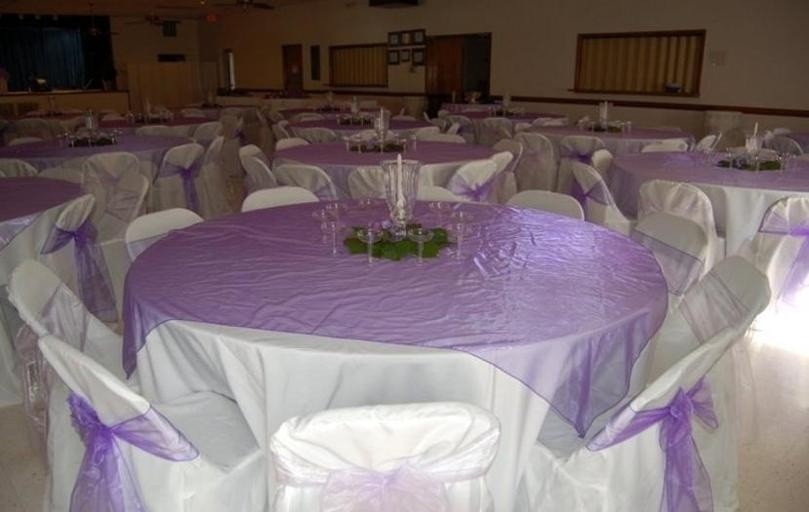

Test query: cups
[382,160,417,242]
[83,111,100,132]
[744,124,765,155]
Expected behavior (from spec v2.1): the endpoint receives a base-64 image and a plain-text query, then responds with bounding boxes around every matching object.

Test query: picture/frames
[386,28,426,66]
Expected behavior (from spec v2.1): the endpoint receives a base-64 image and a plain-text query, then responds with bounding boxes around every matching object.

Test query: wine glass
[725,150,796,172]
[313,195,473,268]
[336,114,371,127]
[125,111,175,124]
[374,109,392,157]
[343,132,418,153]
[57,130,125,148]
[578,102,632,134]
[487,93,526,118]
